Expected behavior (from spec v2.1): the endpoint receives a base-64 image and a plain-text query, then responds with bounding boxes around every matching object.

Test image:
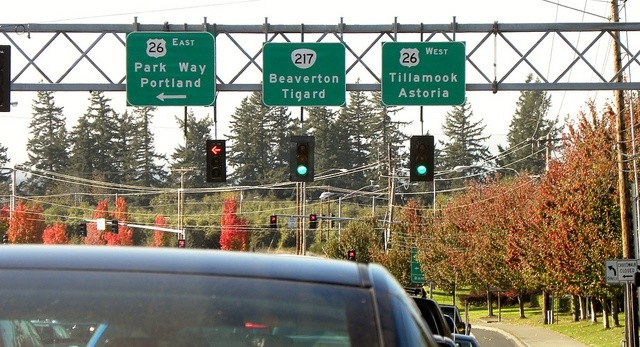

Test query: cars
[437,302,482,347]
[1,243,438,347]
[442,313,480,347]
[407,297,458,347]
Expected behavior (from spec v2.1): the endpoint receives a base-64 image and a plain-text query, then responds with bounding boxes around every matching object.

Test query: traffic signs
[381,41,467,107]
[125,31,217,108]
[618,260,636,281]
[410,246,476,283]
[262,42,348,108]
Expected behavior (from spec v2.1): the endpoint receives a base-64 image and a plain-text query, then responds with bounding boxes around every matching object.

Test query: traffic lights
[269,213,277,228]
[289,133,316,184]
[178,239,185,248]
[78,222,86,238]
[347,250,358,263]
[409,135,436,183]
[309,212,317,228]
[203,138,228,184]
[112,220,119,234]
